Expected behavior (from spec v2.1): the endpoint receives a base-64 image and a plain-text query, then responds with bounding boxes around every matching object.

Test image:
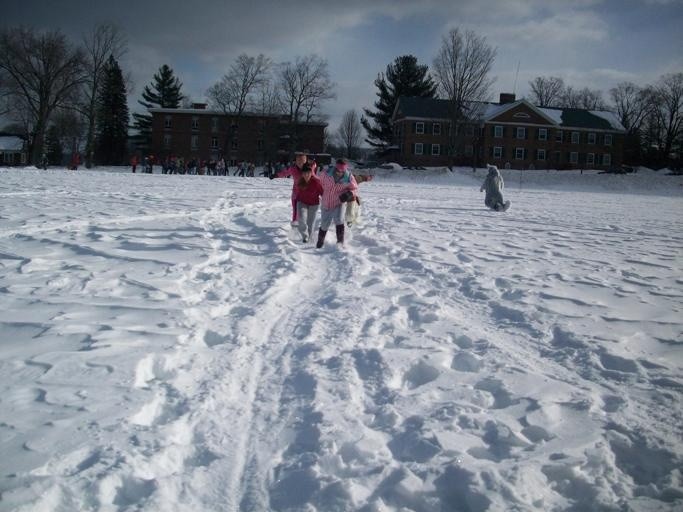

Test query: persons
[479,165,505,211]
[37,153,49,170]
[314,158,360,250]
[291,161,322,245]
[144,155,154,173]
[70,153,80,170]
[260,160,299,178]
[268,152,317,229]
[130,154,137,173]
[161,155,255,177]
[345,201,356,230]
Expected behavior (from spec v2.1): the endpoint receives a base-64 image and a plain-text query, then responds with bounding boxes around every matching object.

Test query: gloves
[339,191,353,202]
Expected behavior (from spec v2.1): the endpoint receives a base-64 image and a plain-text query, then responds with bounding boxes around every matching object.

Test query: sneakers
[303,236,309,242]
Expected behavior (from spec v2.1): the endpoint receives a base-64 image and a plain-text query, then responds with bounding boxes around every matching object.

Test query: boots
[336,224,344,243]
[316,227,327,248]
